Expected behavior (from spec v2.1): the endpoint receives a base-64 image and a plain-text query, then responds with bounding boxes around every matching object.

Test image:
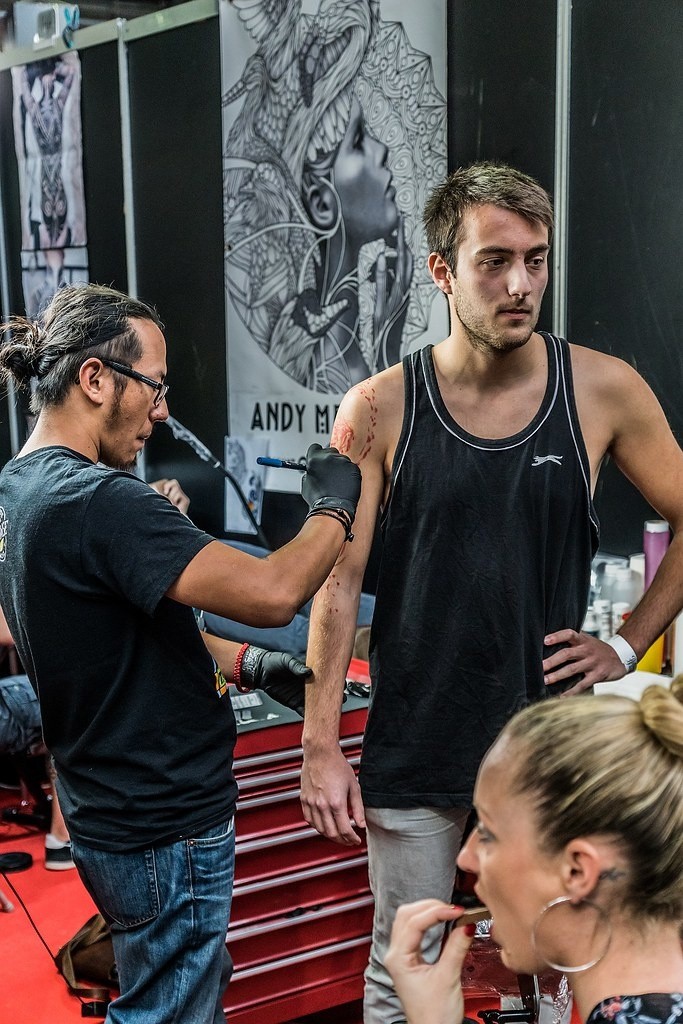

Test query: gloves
[300,443,363,524]
[239,645,348,720]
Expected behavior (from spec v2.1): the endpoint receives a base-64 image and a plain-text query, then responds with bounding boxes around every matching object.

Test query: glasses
[74,360,169,407]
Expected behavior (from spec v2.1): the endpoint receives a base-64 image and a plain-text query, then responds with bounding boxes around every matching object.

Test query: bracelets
[304,508,353,545]
[233,642,250,694]
[608,634,638,674]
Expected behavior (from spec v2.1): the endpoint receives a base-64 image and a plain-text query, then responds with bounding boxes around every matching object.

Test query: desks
[216,658,374,1023]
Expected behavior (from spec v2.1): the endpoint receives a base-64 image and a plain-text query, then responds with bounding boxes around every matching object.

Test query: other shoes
[349,626,371,661]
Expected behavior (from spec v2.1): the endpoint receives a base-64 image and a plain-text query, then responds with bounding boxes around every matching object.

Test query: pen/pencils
[257,457,307,470]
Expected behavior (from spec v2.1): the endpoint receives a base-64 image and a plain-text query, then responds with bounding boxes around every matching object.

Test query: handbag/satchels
[53,924,119,986]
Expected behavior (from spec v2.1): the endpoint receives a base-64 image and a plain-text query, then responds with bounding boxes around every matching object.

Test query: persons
[306,92,414,395]
[0,612,76,870]
[18,58,73,247]
[385,663,683,1023]
[162,477,190,519]
[1,283,359,1024]
[299,166,682,1024]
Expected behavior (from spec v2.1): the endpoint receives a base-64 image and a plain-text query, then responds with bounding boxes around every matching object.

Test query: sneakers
[44,833,77,871]
